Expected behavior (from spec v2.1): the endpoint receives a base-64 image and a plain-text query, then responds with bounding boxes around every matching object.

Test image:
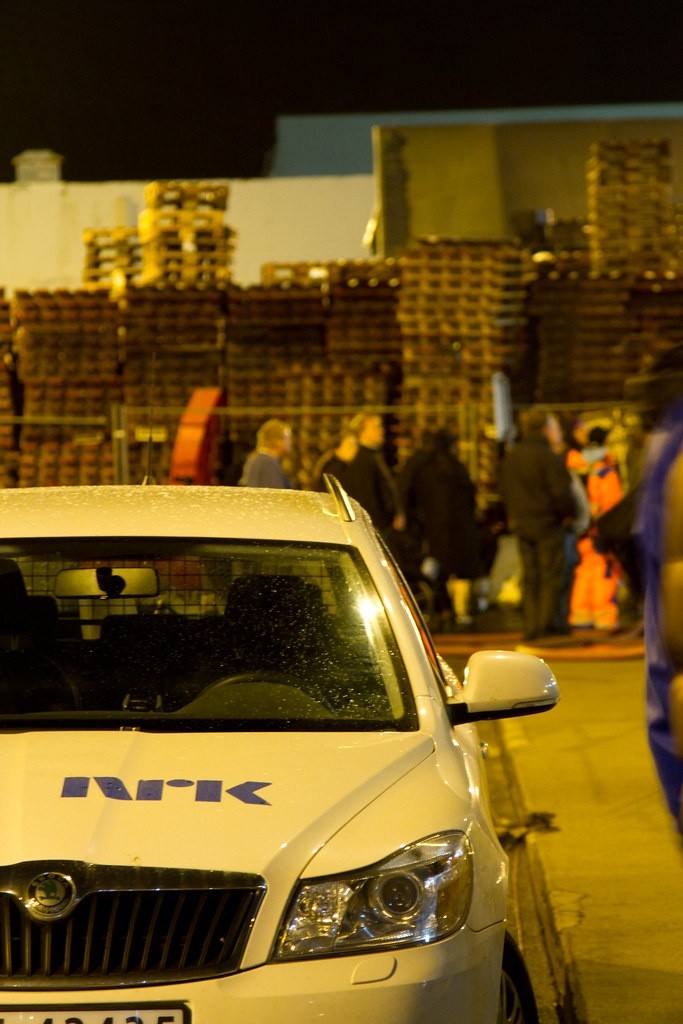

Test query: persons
[333,402,683,641]
[239,420,293,491]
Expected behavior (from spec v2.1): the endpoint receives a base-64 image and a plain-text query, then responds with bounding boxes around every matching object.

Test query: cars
[1,473,562,1024]
